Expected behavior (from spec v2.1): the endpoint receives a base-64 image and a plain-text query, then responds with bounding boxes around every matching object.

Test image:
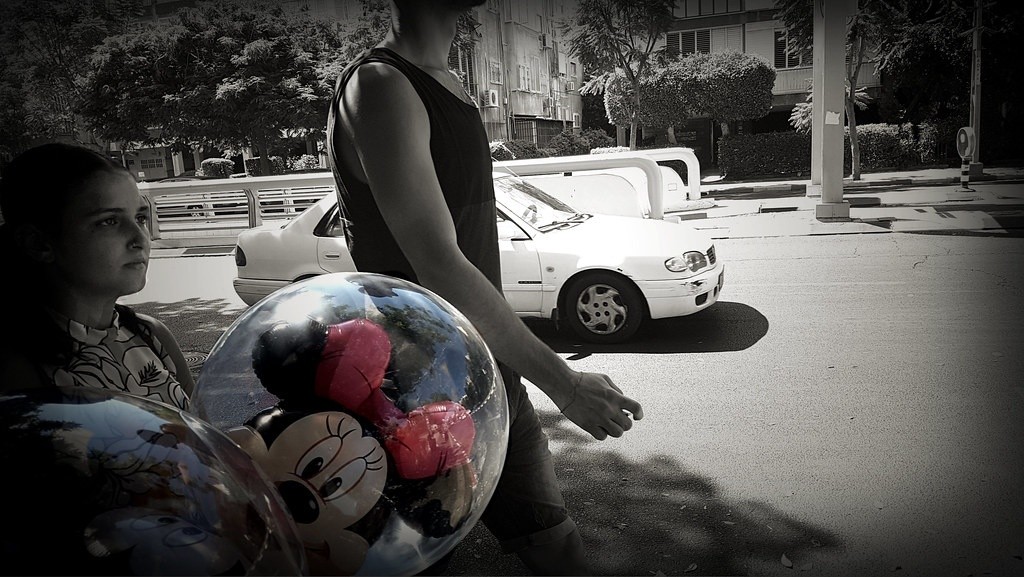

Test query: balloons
[187,271,509,577]
[0,385,309,577]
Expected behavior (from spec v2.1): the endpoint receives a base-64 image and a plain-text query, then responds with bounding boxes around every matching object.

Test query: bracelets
[560,371,583,413]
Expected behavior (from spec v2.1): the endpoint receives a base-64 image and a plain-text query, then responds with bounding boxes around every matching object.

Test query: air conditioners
[484,89,499,106]
[567,82,574,90]
[541,33,552,47]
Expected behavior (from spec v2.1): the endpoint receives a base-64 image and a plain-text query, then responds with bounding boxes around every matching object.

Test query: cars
[153,178,238,217]
[232,173,725,344]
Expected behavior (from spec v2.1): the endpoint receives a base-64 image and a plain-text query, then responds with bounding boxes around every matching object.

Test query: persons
[325,0,644,577]
[0,144,211,577]
